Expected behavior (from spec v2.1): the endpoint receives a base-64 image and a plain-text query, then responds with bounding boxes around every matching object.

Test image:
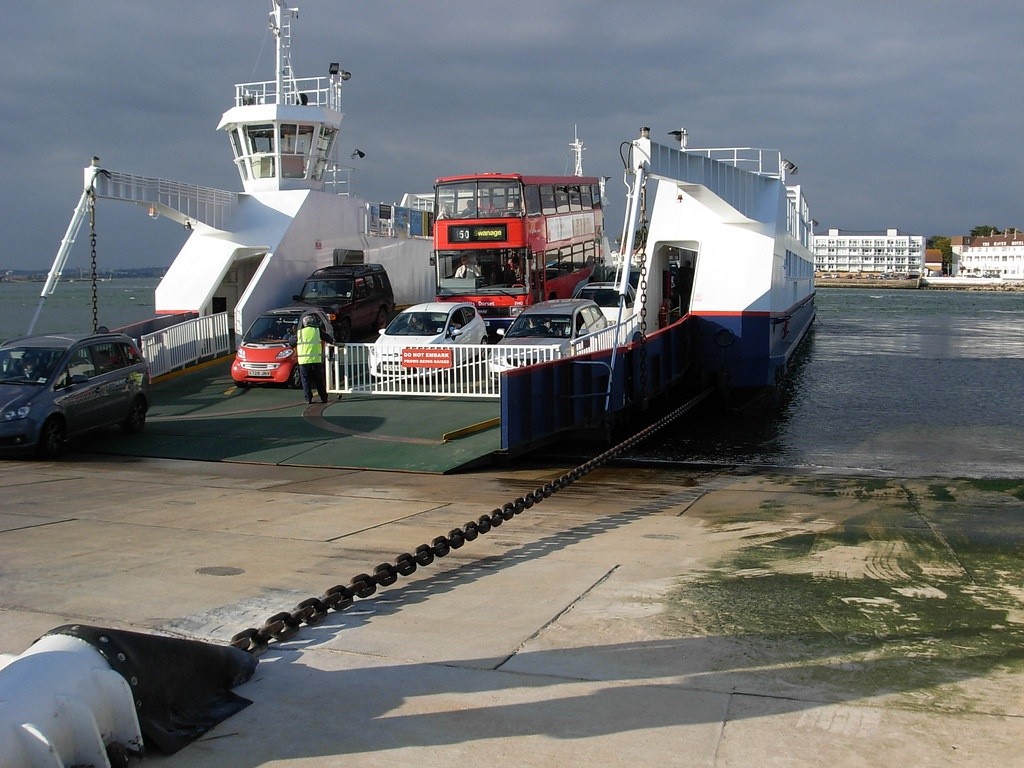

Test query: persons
[287,325,297,337]
[446,318,461,329]
[463,200,481,218]
[511,318,547,336]
[289,315,333,404]
[501,264,516,284]
[454,255,480,278]
[552,321,578,337]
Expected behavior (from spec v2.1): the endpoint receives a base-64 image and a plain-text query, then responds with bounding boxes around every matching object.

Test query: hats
[303,316,313,326]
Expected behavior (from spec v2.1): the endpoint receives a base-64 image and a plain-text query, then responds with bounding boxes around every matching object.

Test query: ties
[463,266,467,278]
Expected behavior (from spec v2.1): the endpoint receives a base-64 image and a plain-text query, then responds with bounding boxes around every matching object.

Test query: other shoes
[322,399,327,403]
[304,400,310,404]
